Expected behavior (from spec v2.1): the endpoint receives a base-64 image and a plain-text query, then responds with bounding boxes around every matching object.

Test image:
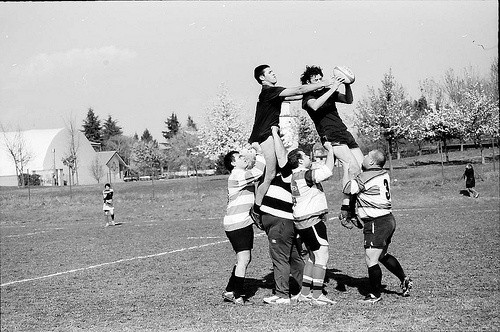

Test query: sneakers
[361,293,383,303]
[400,277,413,297]
[232,296,254,306]
[311,293,337,306]
[221,289,234,301]
[106,223,109,227]
[296,293,312,302]
[249,207,264,230]
[349,213,364,229]
[263,295,291,305]
[111,221,115,226]
[338,214,354,229]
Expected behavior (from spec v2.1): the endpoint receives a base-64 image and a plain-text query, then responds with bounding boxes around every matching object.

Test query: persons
[257,126,306,305]
[287,141,337,307]
[103,183,115,227]
[222,142,266,307]
[248,64,339,231]
[338,150,413,304]
[299,64,364,229]
[463,164,480,198]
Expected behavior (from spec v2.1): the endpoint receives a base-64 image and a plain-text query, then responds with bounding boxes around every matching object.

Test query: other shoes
[475,194,480,198]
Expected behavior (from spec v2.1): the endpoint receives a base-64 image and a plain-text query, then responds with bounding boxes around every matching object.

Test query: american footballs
[333,65,355,84]
